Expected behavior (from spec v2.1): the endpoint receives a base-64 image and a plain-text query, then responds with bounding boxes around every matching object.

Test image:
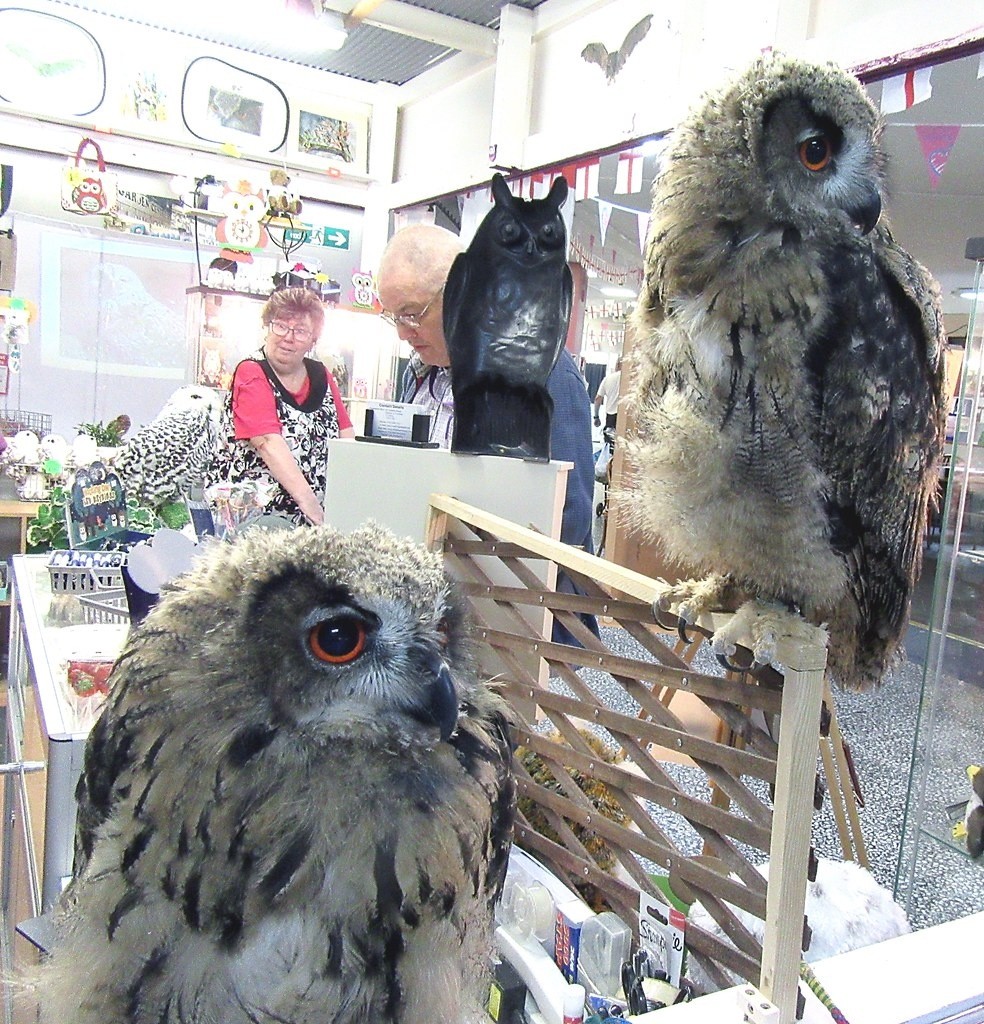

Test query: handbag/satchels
[62,139,117,217]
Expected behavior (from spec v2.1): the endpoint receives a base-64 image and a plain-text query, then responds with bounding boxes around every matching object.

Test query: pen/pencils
[673,986,692,1005]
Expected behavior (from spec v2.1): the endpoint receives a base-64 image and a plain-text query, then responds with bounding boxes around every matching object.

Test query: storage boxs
[75,589,132,626]
[46,549,124,594]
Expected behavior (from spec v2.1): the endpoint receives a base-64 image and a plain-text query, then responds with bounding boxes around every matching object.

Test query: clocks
[213,191,269,248]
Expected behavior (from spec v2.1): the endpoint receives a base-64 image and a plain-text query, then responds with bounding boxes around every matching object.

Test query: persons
[593,357,623,454]
[379,225,603,673]
[219,288,356,528]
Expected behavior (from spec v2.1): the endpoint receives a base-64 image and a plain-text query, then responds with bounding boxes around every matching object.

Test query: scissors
[620,961,648,1016]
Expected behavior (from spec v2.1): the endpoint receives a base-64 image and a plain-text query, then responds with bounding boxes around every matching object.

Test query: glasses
[380,288,443,327]
[270,320,312,342]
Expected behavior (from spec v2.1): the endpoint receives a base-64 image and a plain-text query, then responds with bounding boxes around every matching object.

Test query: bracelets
[594,416,599,419]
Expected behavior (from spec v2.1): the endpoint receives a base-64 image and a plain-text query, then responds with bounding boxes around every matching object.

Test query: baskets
[48,549,124,595]
[79,587,130,625]
